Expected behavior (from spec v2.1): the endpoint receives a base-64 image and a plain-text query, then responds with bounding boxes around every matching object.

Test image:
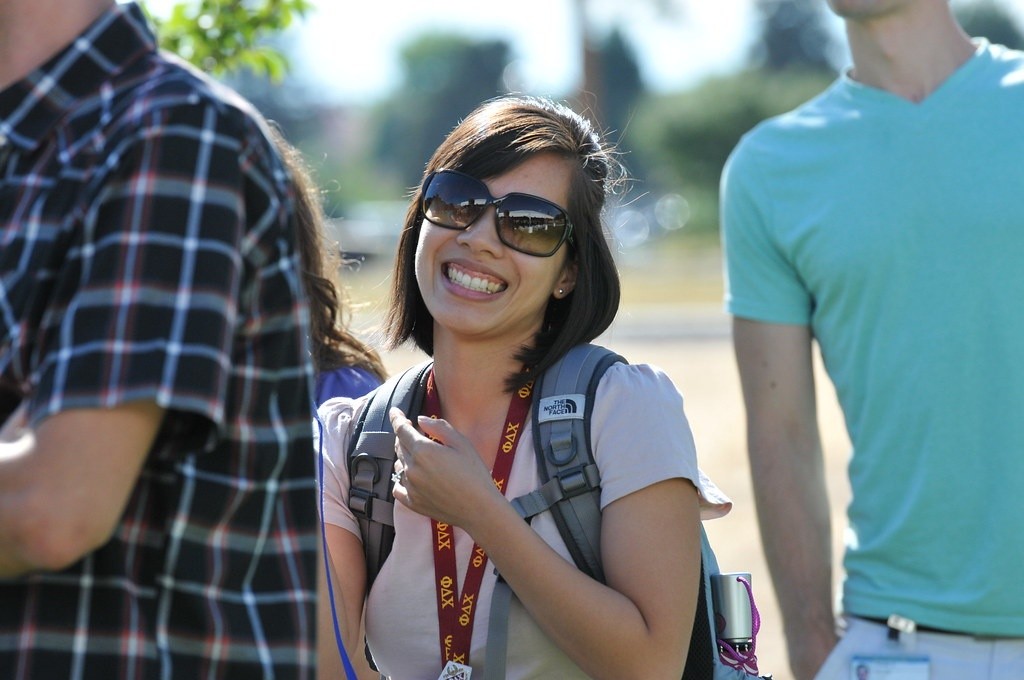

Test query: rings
[390,469,406,483]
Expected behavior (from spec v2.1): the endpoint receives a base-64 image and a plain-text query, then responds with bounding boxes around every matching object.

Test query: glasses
[419,168,574,257]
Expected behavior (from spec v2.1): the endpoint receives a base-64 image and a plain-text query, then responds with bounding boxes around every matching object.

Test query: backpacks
[349,344,762,680]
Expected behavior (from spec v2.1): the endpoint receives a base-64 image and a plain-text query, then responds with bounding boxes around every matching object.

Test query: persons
[0,0,313,679]
[856,664,869,680]
[261,120,387,409]
[720,0,1023,680]
[309,93,734,680]
